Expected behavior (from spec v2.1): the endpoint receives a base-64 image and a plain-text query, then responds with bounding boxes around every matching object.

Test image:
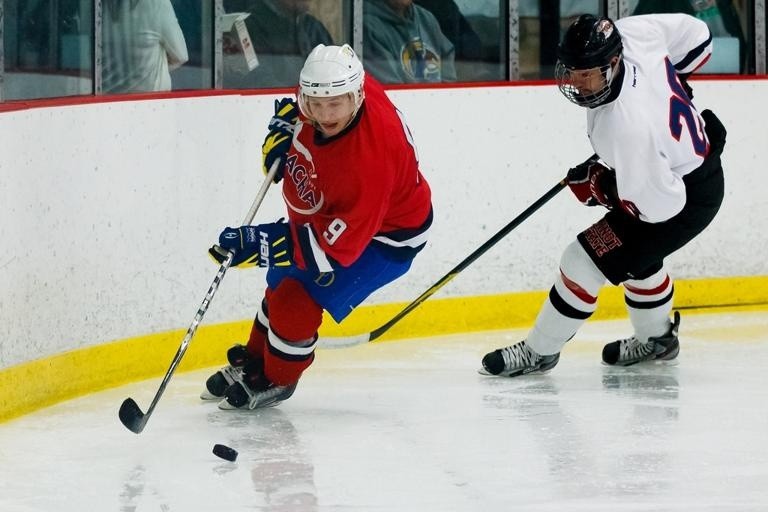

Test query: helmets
[299,44,364,108]
[556,14,623,108]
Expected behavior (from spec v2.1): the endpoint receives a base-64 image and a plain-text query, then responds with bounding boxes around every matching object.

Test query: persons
[475,11,723,371]
[199,42,434,414]
[227,0,334,91]
[356,1,456,85]
[81,2,187,93]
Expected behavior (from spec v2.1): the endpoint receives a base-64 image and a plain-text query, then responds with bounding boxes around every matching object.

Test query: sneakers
[225,364,297,408]
[207,346,257,395]
[483,342,562,376]
[602,312,682,366]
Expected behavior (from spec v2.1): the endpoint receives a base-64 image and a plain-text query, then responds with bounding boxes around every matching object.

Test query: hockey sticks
[120,156,280,434]
[314,153,599,349]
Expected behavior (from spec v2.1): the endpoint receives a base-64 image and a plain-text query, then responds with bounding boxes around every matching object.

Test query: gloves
[262,99,297,184]
[208,217,295,269]
[569,163,621,210]
